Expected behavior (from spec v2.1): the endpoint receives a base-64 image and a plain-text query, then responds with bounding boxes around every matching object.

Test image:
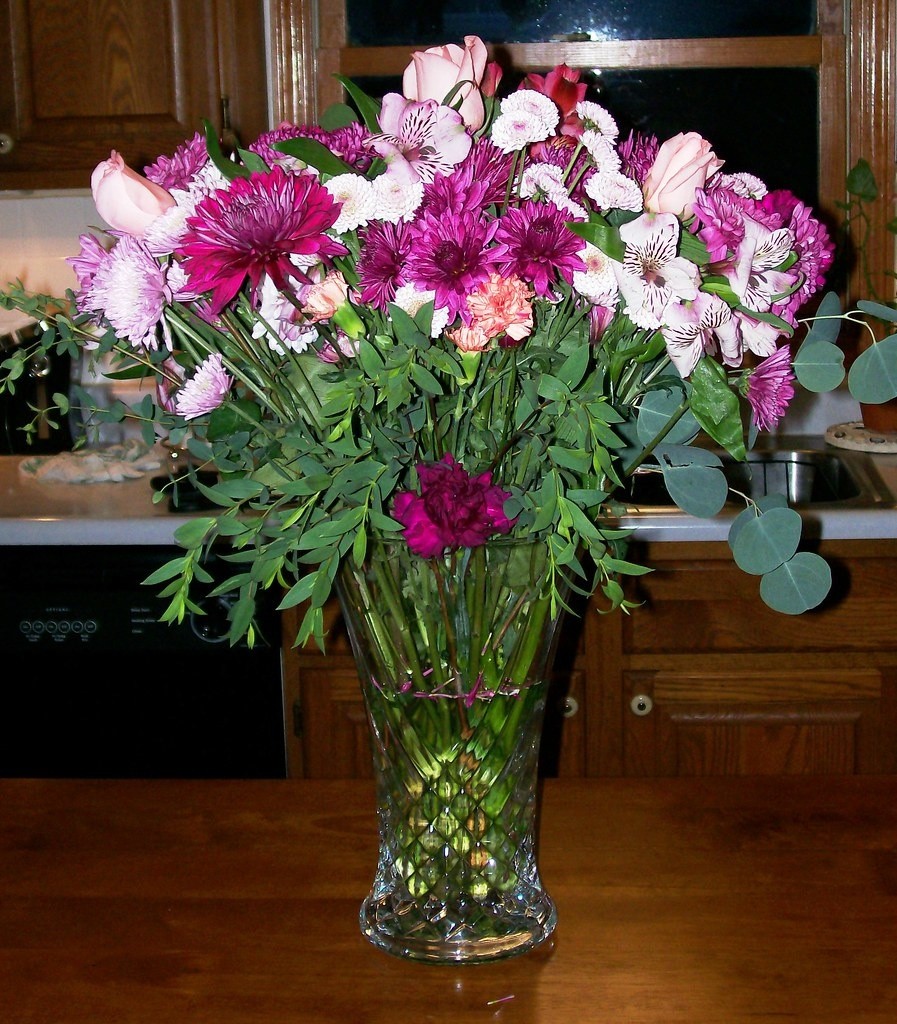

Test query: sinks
[612,430,894,512]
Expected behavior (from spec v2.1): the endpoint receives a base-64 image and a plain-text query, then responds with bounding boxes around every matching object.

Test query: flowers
[1,28,897,941]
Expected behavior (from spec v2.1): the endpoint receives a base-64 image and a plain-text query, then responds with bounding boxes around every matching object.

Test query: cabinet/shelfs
[287,538,897,787]
[0,1,269,191]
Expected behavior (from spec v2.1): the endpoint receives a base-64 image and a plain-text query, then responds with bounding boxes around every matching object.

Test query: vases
[326,535,573,965]
